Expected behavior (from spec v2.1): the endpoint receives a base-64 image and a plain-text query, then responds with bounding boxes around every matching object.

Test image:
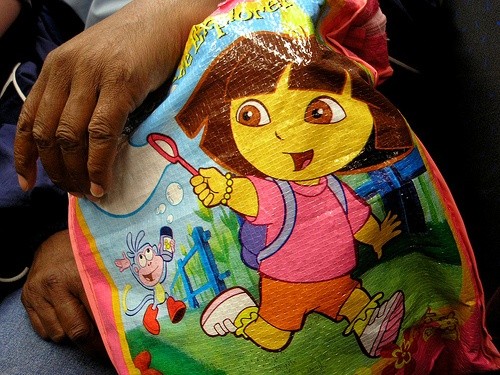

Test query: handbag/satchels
[66,0,500,375]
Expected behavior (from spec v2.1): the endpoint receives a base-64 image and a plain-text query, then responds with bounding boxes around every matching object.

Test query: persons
[0,0,227,348]
